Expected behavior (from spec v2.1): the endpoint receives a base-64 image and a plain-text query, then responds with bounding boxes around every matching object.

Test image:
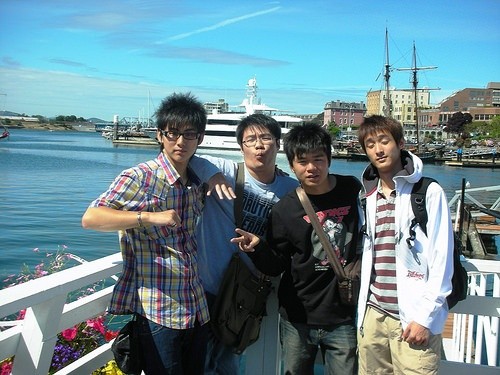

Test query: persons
[124,128,130,140]
[354,115,454,375]
[492,145,498,164]
[230,120,364,375]
[456,146,462,162]
[82,92,300,375]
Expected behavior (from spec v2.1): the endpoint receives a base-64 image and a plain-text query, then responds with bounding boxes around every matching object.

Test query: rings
[171,221,178,227]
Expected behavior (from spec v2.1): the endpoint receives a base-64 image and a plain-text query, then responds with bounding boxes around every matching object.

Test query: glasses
[159,128,203,139]
[243,136,277,147]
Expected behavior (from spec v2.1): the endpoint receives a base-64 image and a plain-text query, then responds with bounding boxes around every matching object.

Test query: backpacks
[362,176,468,311]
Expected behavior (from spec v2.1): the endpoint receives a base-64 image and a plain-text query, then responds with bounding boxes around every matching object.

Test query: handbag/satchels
[339,253,362,302]
[111,320,143,375]
[218,251,272,354]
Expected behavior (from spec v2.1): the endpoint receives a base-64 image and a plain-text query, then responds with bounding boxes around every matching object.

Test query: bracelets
[137,211,142,228]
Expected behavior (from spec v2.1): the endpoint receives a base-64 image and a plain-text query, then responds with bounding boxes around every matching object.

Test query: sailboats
[332,25,446,160]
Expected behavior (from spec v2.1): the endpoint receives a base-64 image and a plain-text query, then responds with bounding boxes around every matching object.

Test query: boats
[446,177,500,298]
[195,75,305,153]
